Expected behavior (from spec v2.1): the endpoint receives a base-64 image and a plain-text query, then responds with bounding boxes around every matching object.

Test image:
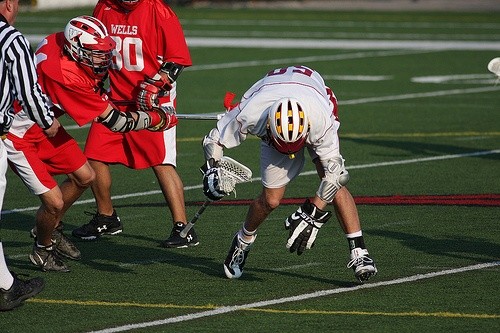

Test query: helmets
[267,96,310,154]
[64,16,115,68]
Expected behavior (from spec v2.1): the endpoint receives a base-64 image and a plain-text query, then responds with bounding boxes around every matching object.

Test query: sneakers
[29,237,70,272]
[161,221,200,249]
[72,209,122,240]
[224,230,257,279]
[347,247,378,282]
[0,272,45,310]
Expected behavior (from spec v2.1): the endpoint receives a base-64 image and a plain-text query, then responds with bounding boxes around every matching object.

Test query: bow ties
[30,226,82,260]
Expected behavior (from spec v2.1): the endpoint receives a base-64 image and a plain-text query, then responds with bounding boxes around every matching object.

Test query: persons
[71,0,199,247]
[0,0,61,311]
[5,15,177,272]
[201,65,379,283]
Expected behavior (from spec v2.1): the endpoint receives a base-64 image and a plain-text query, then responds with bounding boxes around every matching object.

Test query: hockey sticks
[487,58,500,78]
[173,110,228,121]
[178,156,254,243]
[112,99,159,106]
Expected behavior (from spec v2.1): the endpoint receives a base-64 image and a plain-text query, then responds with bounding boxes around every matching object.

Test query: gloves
[136,73,172,110]
[148,106,178,131]
[199,157,226,202]
[284,198,332,256]
